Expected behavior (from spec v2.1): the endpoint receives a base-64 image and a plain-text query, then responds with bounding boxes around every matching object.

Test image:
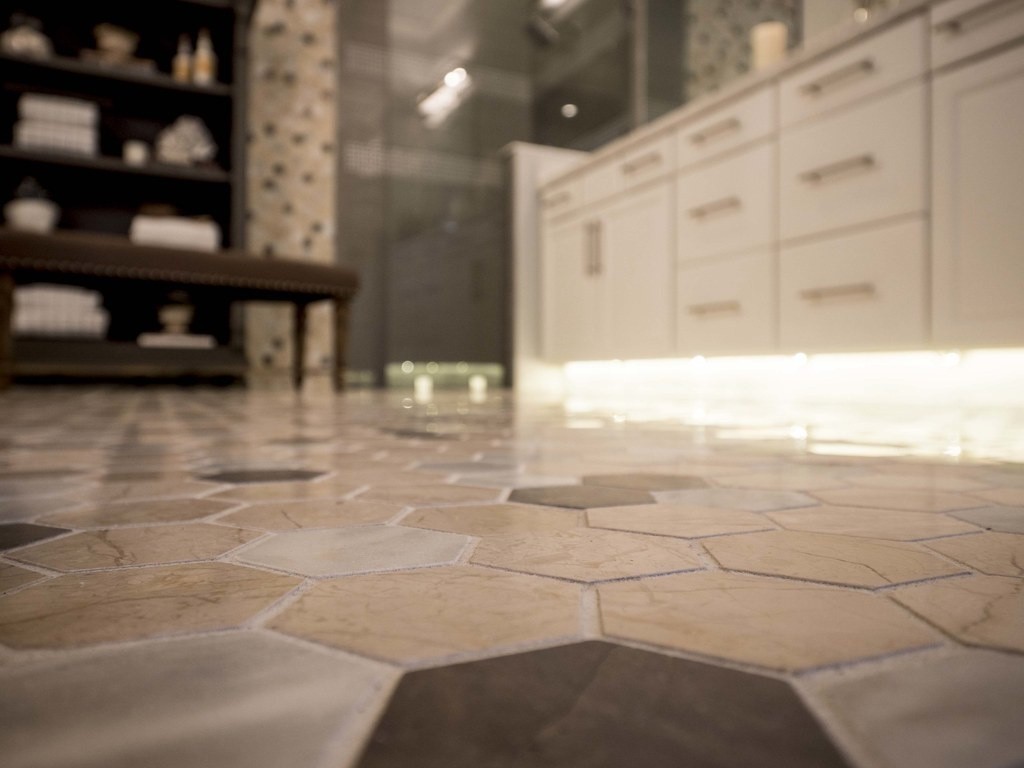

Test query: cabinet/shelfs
[539,0,1024,362]
[0,0,263,388]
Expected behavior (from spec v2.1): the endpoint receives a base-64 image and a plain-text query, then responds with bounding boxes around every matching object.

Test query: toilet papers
[748,20,789,70]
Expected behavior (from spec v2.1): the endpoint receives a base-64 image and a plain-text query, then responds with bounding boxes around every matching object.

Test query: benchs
[0,226,360,395]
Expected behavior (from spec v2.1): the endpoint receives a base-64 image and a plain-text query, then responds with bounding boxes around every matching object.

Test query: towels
[11,285,108,337]
[127,215,221,254]
[13,93,100,157]
